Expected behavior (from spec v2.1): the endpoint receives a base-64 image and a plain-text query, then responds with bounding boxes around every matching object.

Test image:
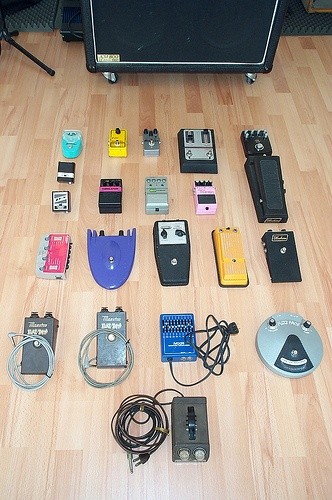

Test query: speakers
[80,0,291,73]
